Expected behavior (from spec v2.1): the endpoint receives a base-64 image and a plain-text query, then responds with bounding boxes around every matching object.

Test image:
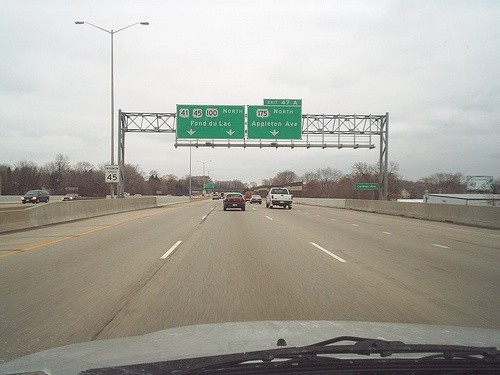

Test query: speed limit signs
[104,165,119,183]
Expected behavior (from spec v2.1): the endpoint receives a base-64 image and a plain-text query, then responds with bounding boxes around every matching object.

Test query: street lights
[75,21,149,199]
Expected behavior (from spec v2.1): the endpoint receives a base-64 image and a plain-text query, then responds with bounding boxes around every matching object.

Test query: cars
[250,195,263,204]
[223,192,246,211]
[21,189,50,204]
[62,193,82,202]
[212,193,220,201]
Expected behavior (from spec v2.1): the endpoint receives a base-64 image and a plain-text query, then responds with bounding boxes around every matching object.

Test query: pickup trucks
[265,186,294,208]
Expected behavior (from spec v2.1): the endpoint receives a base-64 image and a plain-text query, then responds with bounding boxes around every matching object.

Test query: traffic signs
[247,104,306,141]
[175,103,245,140]
